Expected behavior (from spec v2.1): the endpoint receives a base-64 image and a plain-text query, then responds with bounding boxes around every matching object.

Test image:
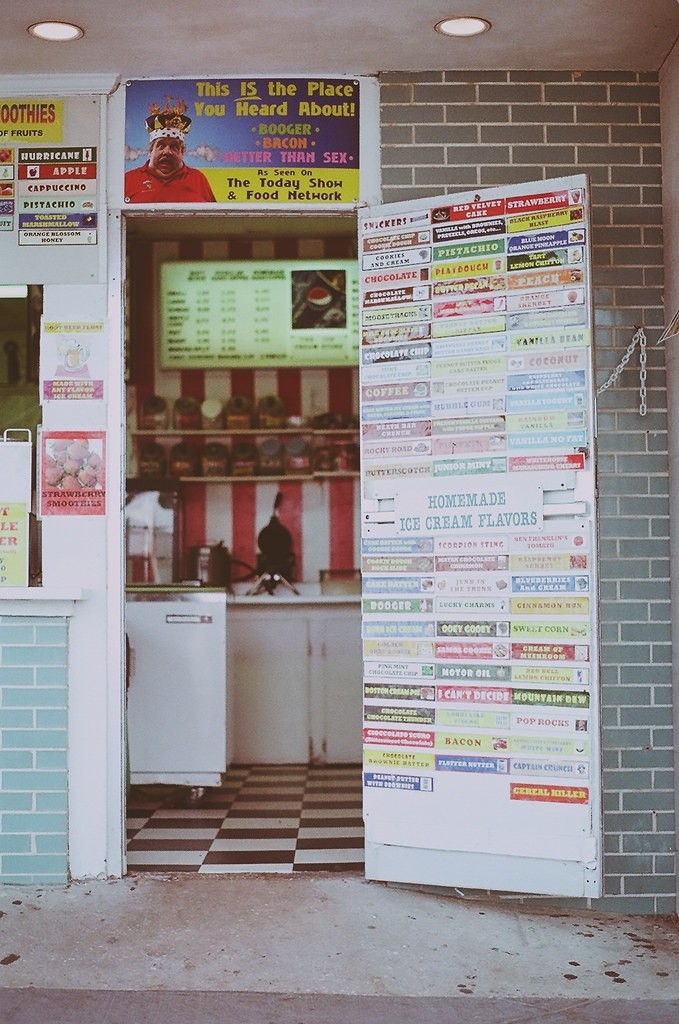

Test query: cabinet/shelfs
[231,606,362,766]
[130,428,361,483]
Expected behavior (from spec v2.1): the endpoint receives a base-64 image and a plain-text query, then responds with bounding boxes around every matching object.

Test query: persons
[125,95,217,202]
